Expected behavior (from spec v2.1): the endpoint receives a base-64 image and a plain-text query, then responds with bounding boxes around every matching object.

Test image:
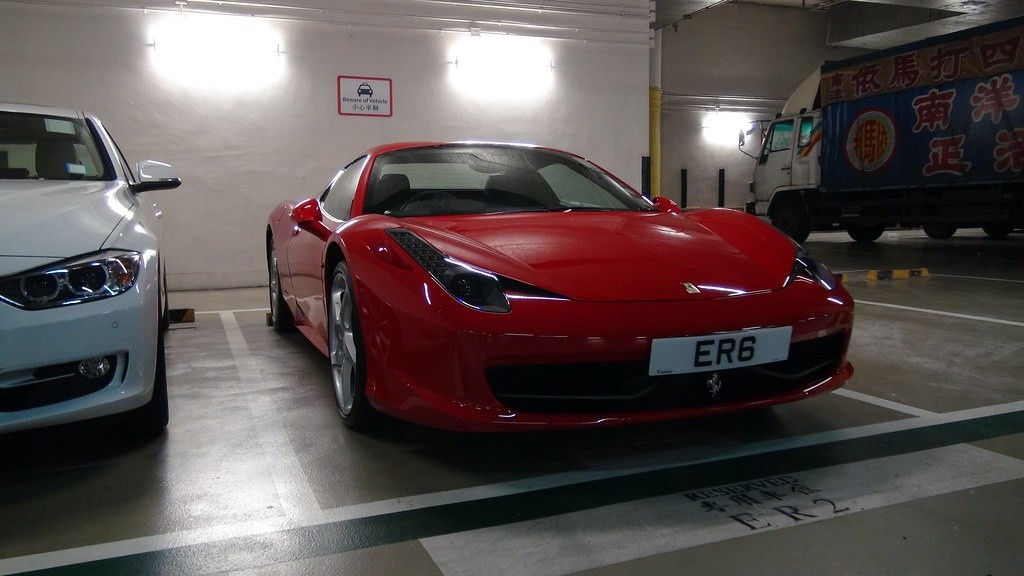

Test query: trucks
[735,16,1024,247]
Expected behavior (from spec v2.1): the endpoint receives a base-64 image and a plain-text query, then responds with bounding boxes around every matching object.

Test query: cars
[0,101,181,448]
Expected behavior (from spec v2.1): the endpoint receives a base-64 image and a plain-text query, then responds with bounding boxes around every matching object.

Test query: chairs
[34,138,81,180]
[484,172,532,202]
[373,173,412,213]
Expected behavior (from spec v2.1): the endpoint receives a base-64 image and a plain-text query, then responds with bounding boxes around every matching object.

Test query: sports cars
[263,140,856,438]
